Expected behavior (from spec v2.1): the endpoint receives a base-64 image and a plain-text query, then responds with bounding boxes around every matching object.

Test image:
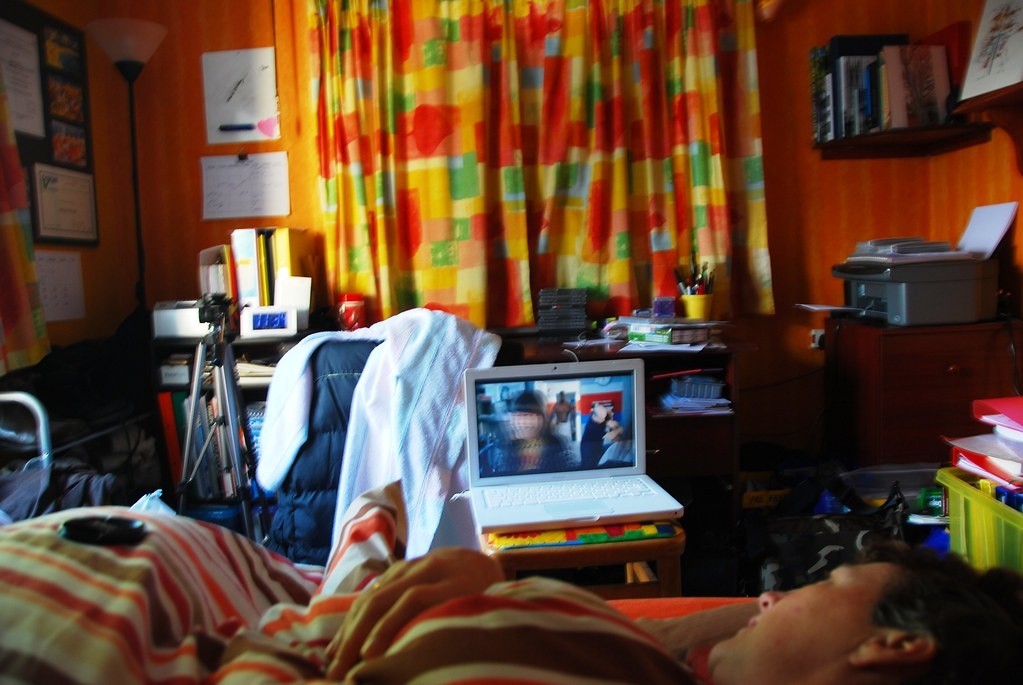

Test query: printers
[827,250,1000,326]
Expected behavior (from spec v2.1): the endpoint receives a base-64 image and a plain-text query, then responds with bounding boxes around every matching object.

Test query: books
[198,225,326,314]
[811,19,952,143]
[157,390,236,501]
[854,237,949,259]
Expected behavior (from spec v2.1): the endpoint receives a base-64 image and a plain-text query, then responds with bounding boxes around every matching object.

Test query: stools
[478,518,686,602]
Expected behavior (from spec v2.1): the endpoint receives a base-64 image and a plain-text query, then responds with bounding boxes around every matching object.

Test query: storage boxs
[154,301,214,339]
[933,461,1023,573]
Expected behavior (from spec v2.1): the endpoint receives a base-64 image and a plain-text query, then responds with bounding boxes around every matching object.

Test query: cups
[681,294,713,322]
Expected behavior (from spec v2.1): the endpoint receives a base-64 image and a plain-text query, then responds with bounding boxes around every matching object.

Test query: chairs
[266,339,390,571]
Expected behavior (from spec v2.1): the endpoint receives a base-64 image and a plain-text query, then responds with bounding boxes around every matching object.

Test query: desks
[821,319,1023,462]
[490,328,739,482]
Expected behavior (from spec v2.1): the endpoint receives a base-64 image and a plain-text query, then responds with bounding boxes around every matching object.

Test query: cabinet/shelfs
[159,332,299,510]
[0,335,167,520]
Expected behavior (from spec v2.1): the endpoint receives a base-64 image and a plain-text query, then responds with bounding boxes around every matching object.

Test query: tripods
[178,292,269,545]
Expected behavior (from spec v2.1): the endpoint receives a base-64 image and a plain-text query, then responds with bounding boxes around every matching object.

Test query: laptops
[464,358,684,535]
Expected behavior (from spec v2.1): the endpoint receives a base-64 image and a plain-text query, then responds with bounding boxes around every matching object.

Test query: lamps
[85,17,168,311]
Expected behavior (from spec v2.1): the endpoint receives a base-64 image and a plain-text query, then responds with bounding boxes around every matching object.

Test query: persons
[504,390,623,476]
[323,545,1023,685]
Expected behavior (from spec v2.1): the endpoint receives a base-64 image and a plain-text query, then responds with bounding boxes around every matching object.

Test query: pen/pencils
[673,250,715,295]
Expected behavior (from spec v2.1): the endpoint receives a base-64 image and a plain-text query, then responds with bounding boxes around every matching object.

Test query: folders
[972,397,1022,430]
[952,446,1023,493]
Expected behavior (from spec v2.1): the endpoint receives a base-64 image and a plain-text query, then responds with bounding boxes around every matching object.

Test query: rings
[372,582,382,589]
